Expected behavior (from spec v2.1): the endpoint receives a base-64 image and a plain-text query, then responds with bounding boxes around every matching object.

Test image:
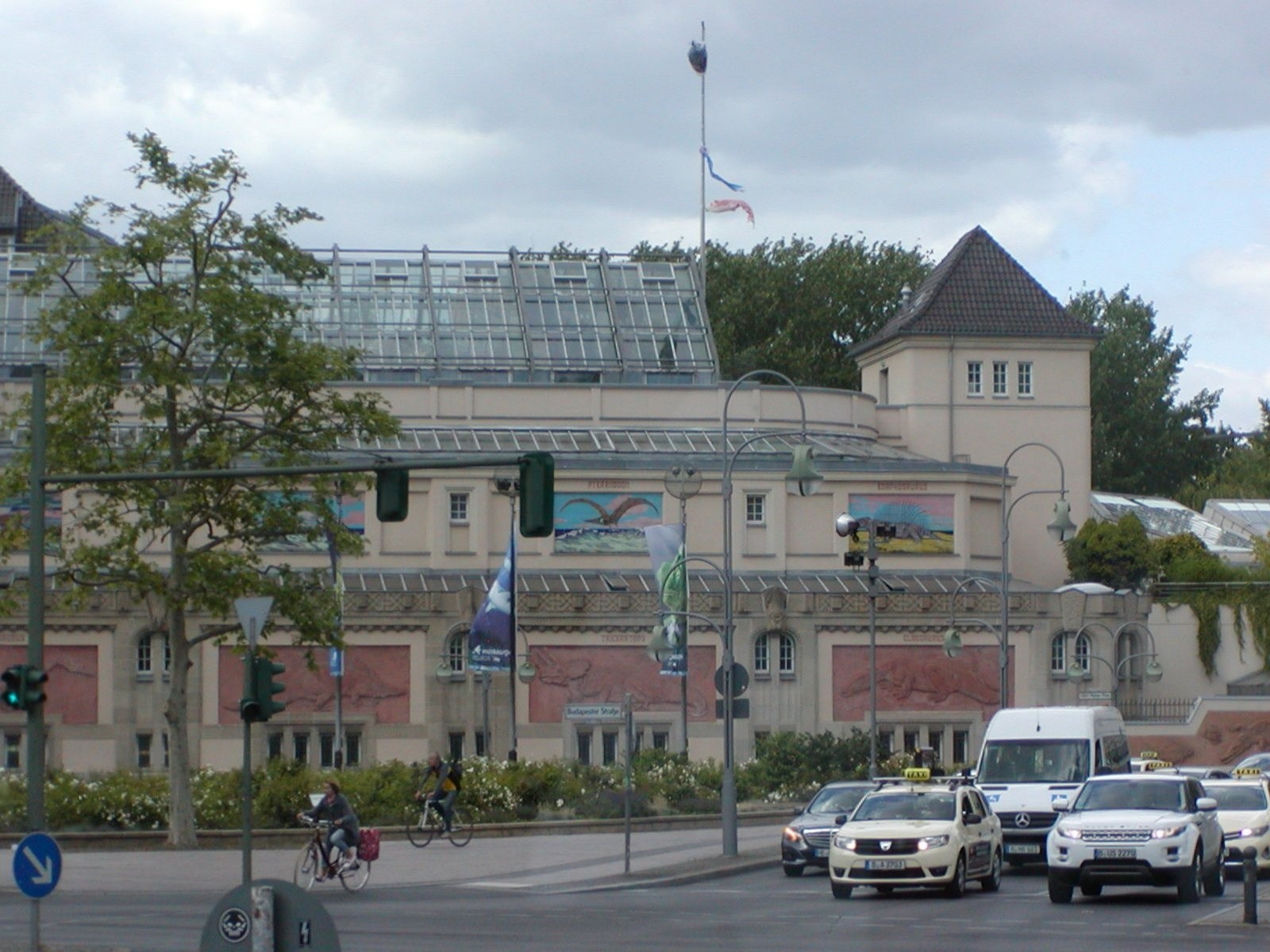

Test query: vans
[960,706,1132,868]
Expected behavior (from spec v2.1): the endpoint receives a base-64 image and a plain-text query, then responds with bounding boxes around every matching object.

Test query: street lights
[943,442,1076,708]
[439,622,537,756]
[663,462,702,760]
[494,467,519,761]
[1068,622,1163,707]
[646,370,825,860]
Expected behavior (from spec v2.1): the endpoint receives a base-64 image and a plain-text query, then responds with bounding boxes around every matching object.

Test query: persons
[867,798,981,824]
[984,747,1083,782]
[297,779,358,882]
[414,752,461,838]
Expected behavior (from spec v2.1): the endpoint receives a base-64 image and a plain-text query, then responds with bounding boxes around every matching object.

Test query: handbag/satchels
[356,827,380,861]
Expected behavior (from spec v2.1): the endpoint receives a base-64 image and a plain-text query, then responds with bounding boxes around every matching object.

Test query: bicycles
[406,793,474,847]
[293,816,371,892]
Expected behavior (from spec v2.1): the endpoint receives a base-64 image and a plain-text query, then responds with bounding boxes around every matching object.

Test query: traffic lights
[239,699,261,723]
[255,659,286,723]
[0,664,49,710]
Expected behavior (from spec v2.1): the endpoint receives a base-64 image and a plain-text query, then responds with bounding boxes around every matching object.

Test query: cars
[1131,751,1270,881]
[829,768,1004,899]
[781,780,893,876]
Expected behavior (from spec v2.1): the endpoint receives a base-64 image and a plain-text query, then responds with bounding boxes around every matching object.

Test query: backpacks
[445,762,462,779]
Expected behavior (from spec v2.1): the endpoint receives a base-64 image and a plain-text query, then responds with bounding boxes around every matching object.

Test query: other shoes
[343,855,356,868]
[440,831,453,838]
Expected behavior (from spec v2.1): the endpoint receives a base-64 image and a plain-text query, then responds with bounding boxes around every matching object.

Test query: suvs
[1046,773,1225,904]
